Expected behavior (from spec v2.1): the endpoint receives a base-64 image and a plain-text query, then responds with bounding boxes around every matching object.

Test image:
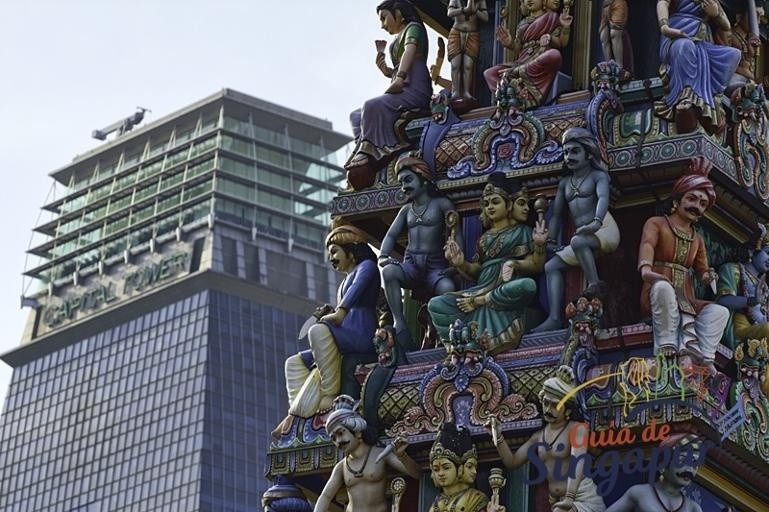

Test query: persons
[258,1,768,511]
